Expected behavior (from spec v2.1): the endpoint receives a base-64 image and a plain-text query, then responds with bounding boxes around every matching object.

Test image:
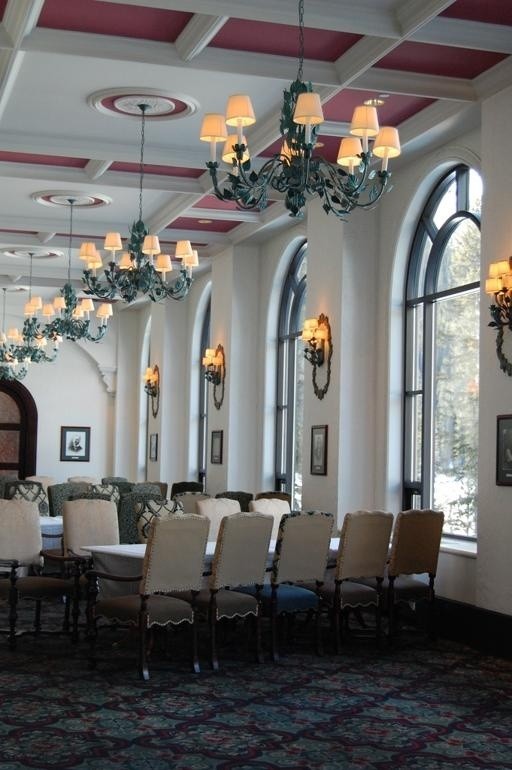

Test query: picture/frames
[60,426,93,462]
[210,430,223,464]
[149,433,157,462]
[309,424,327,475]
[494,412,511,487]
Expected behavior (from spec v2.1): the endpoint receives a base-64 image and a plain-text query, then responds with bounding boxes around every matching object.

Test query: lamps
[0,288,30,383]
[0,253,62,364]
[81,104,199,304]
[201,343,226,410]
[23,199,112,344]
[300,312,334,401]
[483,256,512,378]
[143,364,160,419]
[198,1,403,219]
